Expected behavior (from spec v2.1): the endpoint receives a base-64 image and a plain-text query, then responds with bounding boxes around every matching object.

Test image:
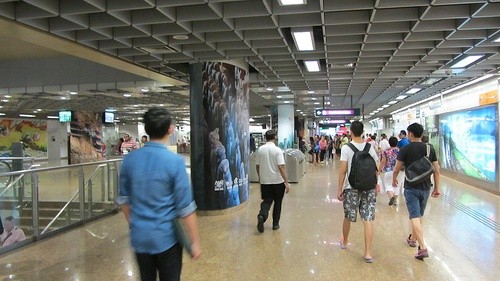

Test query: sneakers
[415,245,429,259]
[407,234,416,247]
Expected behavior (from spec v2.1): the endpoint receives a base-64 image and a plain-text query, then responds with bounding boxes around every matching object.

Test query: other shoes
[389,197,395,205]
[273,225,279,230]
[258,216,264,233]
[339,233,348,248]
[365,256,373,262]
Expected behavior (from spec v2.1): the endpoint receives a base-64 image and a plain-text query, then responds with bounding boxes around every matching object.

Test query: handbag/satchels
[308,150,314,155]
[404,155,435,187]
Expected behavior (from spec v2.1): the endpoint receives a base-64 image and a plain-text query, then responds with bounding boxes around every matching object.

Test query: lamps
[420,78,442,86]
[303,60,321,72]
[278,0,308,6]
[449,54,485,68]
[290,26,316,51]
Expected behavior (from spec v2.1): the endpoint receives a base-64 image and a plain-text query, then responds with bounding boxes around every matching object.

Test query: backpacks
[343,142,378,191]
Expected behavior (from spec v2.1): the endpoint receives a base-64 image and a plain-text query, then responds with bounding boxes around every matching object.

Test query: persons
[421,135,434,186]
[380,136,405,206]
[250,133,256,153]
[255,130,291,233]
[337,121,380,263]
[392,122,441,258]
[118,138,124,156]
[379,134,390,151]
[141,136,148,144]
[309,136,315,163]
[299,136,307,159]
[397,130,409,148]
[119,106,202,281]
[368,136,379,154]
[319,136,327,163]
[0,216,26,248]
[314,135,319,163]
[121,134,138,155]
[208,122,249,208]
[328,136,334,160]
[334,131,372,159]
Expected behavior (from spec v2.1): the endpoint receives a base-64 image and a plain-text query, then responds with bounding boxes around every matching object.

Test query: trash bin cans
[282,149,306,182]
[249,151,259,183]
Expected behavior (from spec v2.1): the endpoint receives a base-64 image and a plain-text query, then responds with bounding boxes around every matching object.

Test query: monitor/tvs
[58,110,73,123]
[104,112,115,123]
